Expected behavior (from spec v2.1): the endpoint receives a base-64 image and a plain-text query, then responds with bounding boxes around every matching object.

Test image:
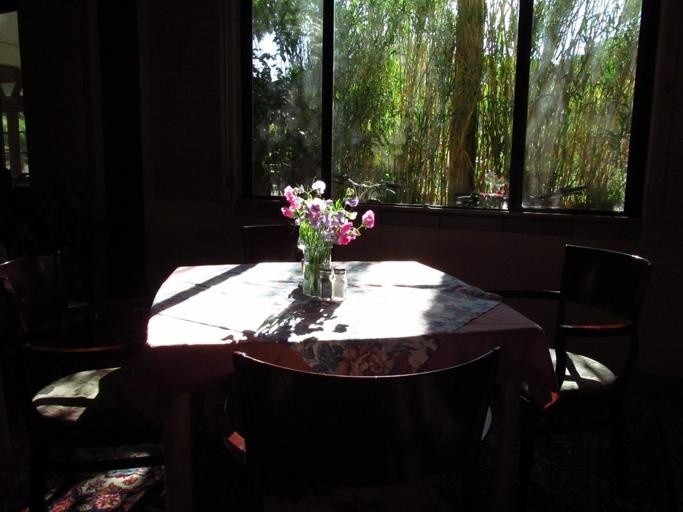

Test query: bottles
[319,268,347,300]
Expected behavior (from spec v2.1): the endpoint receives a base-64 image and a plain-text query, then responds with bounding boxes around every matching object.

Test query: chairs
[1,224,655,511]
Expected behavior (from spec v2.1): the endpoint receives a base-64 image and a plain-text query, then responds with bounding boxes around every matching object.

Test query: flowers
[280,180,375,298]
